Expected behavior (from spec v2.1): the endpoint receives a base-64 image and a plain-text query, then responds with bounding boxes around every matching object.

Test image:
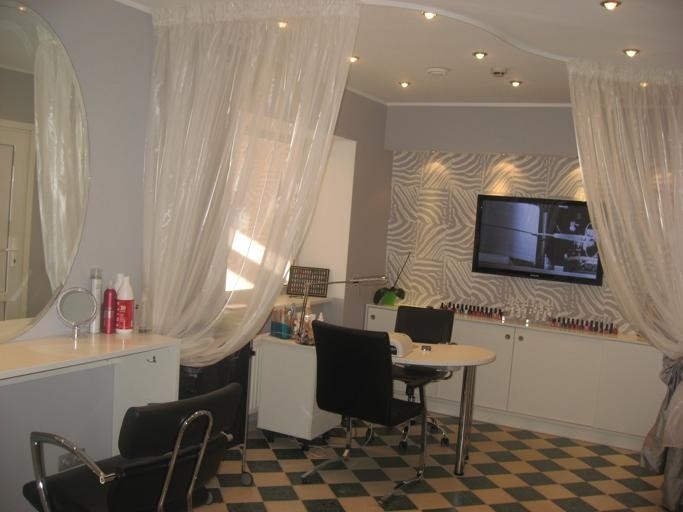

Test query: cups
[139,292,152,335]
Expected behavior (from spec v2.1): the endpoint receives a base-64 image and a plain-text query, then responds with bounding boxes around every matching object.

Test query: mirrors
[1,0,92,347]
[57,289,97,345]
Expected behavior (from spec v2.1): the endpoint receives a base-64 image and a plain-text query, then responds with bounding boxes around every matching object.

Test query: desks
[392,341,494,475]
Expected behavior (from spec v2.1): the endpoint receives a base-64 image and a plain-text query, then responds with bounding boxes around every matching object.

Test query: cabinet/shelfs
[110,346,179,457]
[0,359,113,511]
[363,305,667,452]
[254,336,342,449]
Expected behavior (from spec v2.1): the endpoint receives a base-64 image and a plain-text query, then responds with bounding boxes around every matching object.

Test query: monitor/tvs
[471,193,603,286]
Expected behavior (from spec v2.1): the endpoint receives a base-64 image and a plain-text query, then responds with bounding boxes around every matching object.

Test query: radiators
[250,332,270,417]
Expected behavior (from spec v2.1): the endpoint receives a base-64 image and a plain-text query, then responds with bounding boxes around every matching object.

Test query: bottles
[311,314,317,344]
[115,273,125,292]
[115,275,135,334]
[300,315,312,345]
[317,311,324,322]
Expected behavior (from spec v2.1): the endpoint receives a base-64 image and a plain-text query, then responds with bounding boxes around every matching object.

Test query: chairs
[22,383,244,511]
[366,305,455,451]
[301,321,428,506]
